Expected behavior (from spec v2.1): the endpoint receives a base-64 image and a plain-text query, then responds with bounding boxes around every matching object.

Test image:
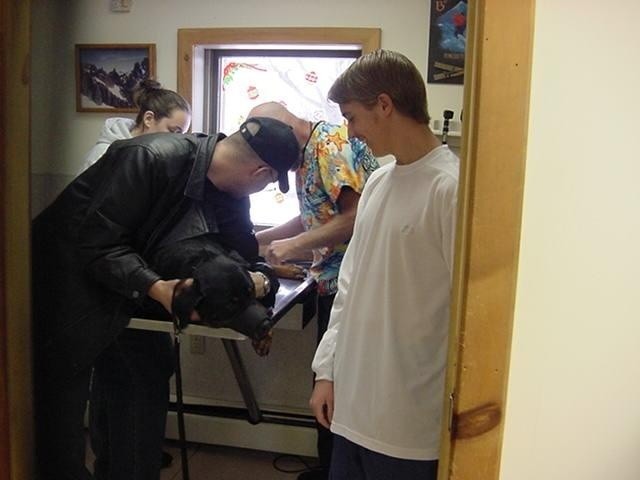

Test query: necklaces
[299,120,326,169]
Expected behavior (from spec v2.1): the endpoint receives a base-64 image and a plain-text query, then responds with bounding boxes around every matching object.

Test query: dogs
[146,238,308,341]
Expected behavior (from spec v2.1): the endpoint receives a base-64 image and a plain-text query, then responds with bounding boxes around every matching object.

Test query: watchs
[254,270,271,301]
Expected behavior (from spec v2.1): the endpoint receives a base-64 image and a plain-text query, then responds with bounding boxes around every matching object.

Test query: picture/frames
[74,41,156,113]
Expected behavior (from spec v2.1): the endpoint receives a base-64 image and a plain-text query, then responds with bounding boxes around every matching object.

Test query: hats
[240,116,299,194]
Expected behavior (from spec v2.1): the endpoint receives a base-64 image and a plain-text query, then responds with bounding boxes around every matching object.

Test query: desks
[122,272,332,478]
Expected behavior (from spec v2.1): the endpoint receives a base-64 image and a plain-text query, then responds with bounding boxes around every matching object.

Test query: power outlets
[190,334,205,354]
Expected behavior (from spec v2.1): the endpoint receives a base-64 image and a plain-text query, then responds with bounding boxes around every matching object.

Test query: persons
[307,46,462,480]
[34,114,301,479]
[74,74,192,469]
[243,100,381,478]
